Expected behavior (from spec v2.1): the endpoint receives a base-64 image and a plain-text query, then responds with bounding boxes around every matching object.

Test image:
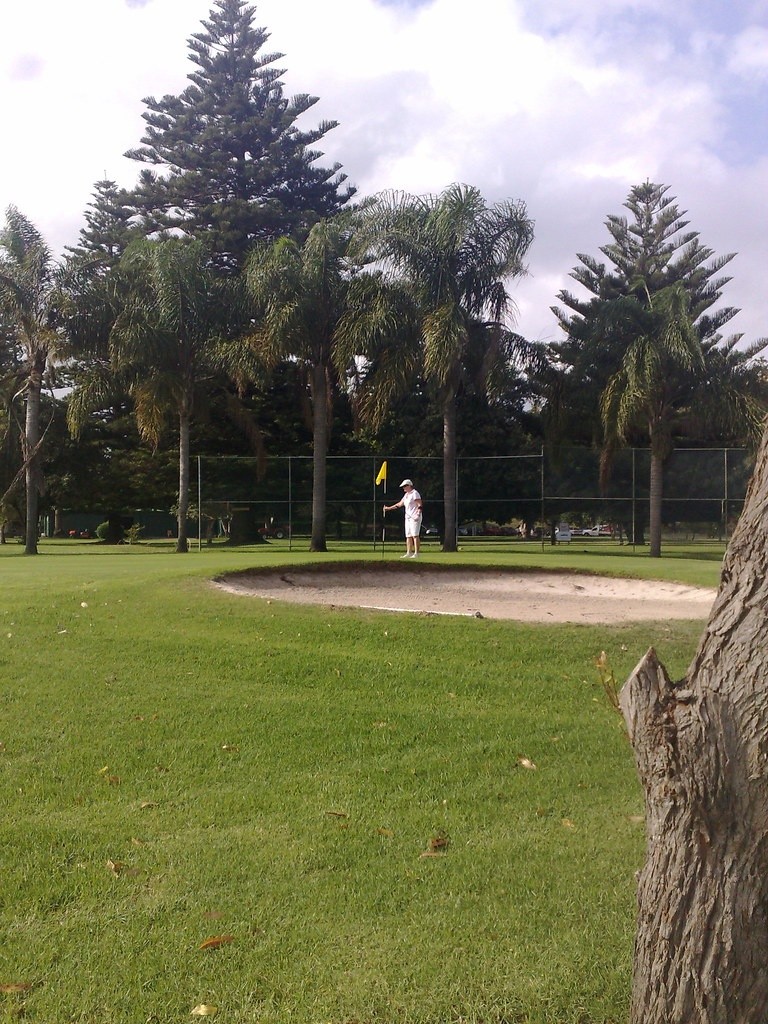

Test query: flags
[376,462,386,485]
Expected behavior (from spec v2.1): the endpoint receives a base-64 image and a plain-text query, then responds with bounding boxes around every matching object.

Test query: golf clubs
[395,505,430,534]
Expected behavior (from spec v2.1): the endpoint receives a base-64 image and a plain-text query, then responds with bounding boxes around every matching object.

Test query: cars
[256,524,291,539]
[570,527,582,536]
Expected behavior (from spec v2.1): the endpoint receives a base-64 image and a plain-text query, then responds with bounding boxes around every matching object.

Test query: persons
[383,480,423,559]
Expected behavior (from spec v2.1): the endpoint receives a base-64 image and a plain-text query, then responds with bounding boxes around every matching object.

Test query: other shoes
[410,553,419,558]
[399,553,413,559]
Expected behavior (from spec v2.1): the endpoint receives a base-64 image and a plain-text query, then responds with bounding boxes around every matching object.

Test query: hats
[399,479,413,487]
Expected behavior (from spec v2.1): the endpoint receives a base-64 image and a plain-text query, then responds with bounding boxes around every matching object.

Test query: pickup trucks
[583,525,614,537]
